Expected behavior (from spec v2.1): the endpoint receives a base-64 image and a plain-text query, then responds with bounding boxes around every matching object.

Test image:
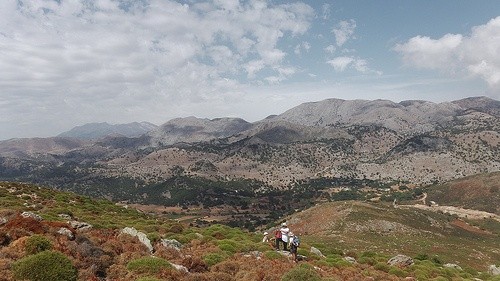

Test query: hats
[264,232,268,236]
[281,223,287,227]
[288,231,294,237]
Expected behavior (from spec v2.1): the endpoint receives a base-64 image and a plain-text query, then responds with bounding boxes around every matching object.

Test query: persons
[263,232,269,242]
[289,231,299,262]
[275,222,290,252]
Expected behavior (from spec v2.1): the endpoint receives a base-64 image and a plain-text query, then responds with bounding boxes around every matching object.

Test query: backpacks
[293,236,301,247]
[274,230,282,238]
